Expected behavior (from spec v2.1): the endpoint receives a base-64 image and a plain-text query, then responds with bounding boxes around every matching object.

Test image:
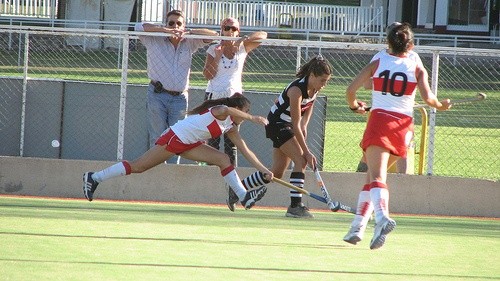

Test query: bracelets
[349,105,360,110]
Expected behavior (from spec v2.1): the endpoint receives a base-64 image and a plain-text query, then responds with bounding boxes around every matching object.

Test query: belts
[150,81,181,95]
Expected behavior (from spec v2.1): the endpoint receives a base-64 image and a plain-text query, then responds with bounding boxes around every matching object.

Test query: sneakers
[240,186,267,210]
[343,225,363,244]
[226,183,238,211]
[370,217,396,249]
[83,171,99,201]
[286,202,314,217]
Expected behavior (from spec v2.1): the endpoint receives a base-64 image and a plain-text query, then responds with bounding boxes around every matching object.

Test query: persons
[203,17,267,167]
[82,92,269,210]
[225,54,332,218]
[356,21,415,174]
[135,10,219,164]
[342,24,452,249]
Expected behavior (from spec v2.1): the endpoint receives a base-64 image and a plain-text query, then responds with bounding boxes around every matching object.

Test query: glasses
[168,21,183,26]
[223,25,238,31]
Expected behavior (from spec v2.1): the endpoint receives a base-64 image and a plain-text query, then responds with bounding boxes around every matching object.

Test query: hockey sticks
[314,163,341,213]
[365,93,487,111]
[264,173,357,215]
